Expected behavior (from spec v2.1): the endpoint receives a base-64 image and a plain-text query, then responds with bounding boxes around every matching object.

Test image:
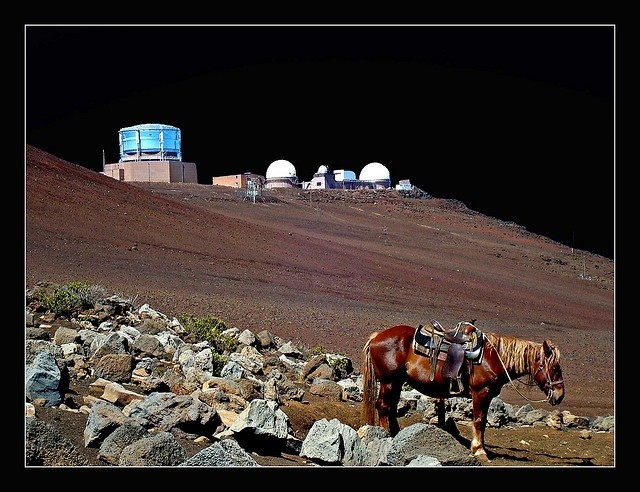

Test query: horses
[359,324,567,465]
